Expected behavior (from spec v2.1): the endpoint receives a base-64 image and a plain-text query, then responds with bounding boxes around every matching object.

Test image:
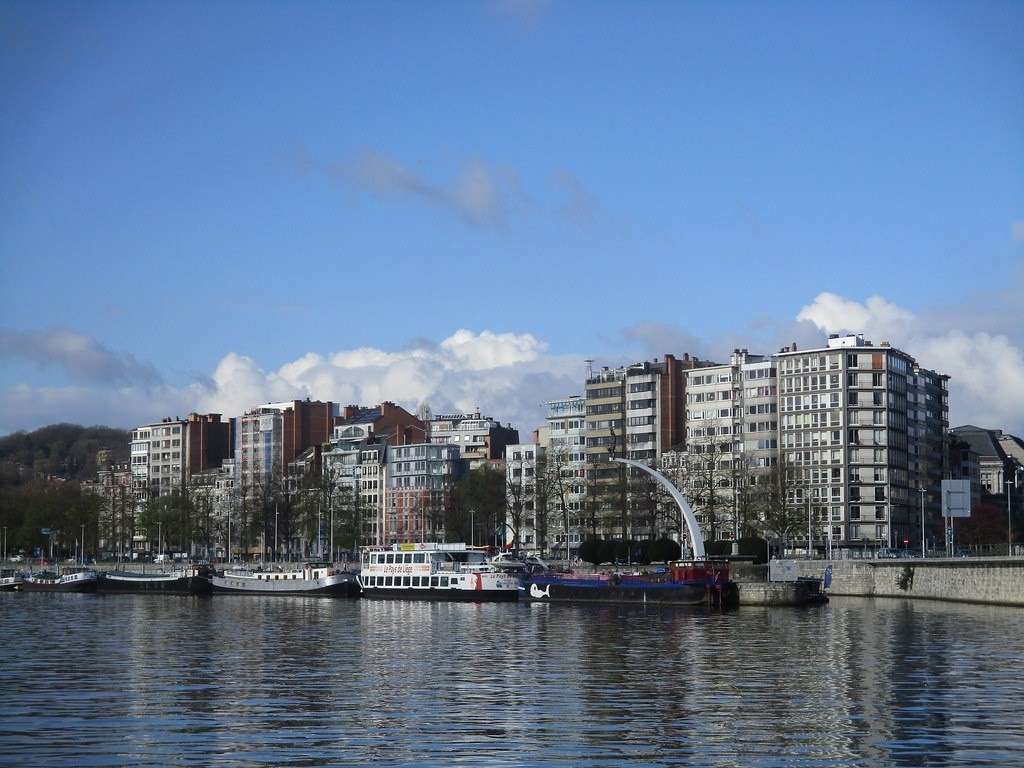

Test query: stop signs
[903,539,908,544]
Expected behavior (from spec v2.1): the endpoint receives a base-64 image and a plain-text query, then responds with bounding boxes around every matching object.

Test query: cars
[133,557,153,564]
[10,554,23,562]
[103,556,129,563]
[32,559,52,566]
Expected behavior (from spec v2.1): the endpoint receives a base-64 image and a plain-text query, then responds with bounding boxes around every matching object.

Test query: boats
[0,566,23,592]
[356,542,519,602]
[738,574,830,606]
[23,566,98,593]
[518,554,731,606]
[98,565,209,595]
[212,562,361,597]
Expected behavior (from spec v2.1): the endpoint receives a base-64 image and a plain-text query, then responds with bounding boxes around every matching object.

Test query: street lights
[3,527,8,562]
[156,521,162,562]
[80,524,85,565]
[918,487,928,558]
[469,509,475,549]
[1004,480,1014,555]
